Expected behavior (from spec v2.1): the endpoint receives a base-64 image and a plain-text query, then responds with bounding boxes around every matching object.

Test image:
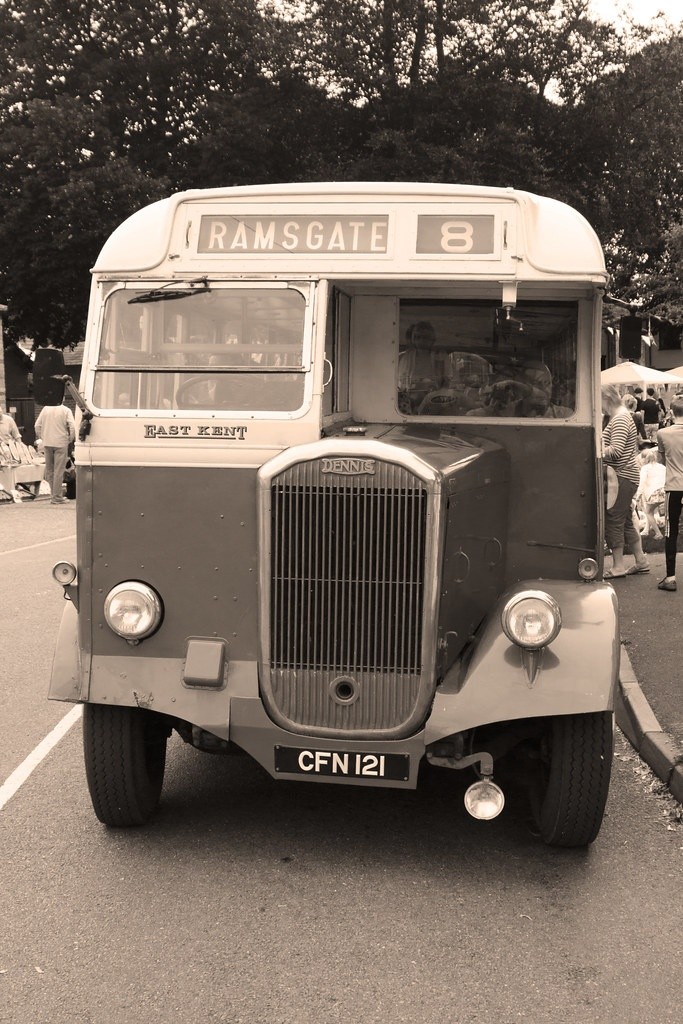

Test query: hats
[444,352,495,375]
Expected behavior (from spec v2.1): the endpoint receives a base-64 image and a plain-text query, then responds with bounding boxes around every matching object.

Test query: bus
[47,181,623,846]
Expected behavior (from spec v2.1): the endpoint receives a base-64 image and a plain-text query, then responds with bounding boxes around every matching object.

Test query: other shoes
[50,497,70,505]
[657,577,676,592]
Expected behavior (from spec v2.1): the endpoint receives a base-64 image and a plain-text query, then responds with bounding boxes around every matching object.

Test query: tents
[600,362,683,424]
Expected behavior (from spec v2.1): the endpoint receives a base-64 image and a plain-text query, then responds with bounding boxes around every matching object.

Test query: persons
[398,319,452,402]
[657,398,683,591]
[622,387,667,440]
[511,360,574,418]
[35,390,75,504]
[601,384,651,579]
[0,406,22,445]
[636,449,666,540]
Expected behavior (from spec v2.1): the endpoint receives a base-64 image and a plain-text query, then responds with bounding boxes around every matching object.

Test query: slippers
[603,568,626,578]
[625,562,650,575]
